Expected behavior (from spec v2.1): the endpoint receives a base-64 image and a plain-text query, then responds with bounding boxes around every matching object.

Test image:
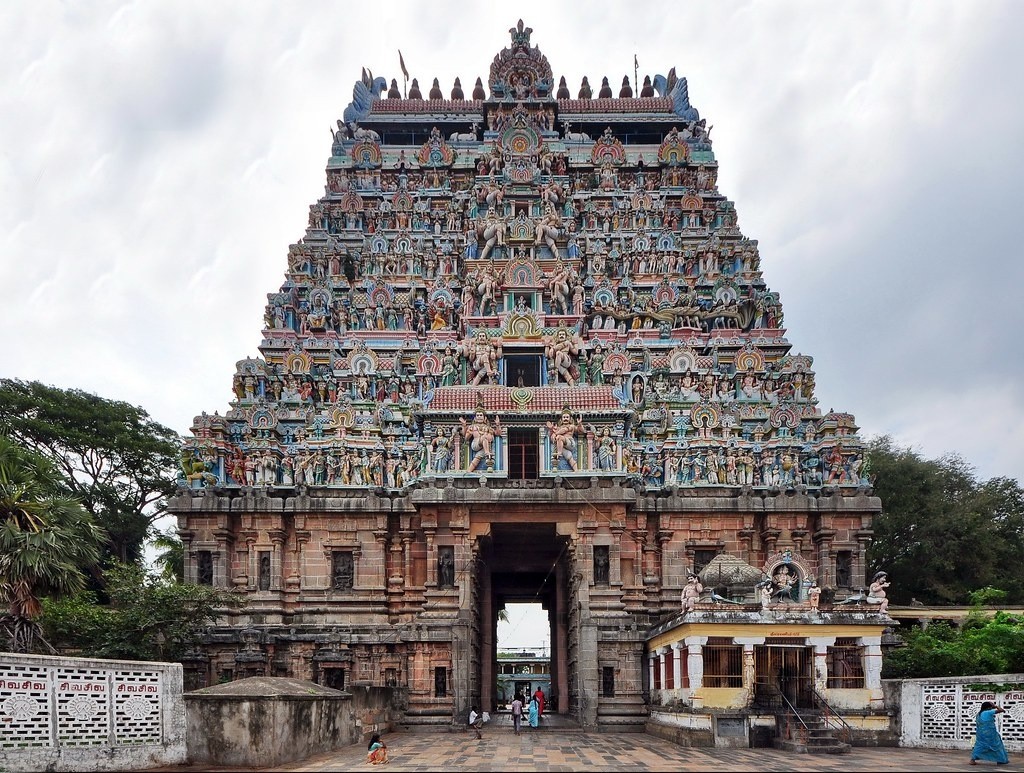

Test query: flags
[400,52,409,81]
[635,58,639,69]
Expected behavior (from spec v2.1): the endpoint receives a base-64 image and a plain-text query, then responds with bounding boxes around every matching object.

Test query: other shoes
[530,724,538,728]
[540,715,542,718]
[514,732,520,736]
[476,726,482,729]
[538,714,539,715]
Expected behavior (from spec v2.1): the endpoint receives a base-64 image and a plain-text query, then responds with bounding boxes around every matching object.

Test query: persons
[528,695,539,730]
[761,579,773,611]
[836,556,852,586]
[437,671,445,694]
[535,686,547,718]
[773,567,798,604]
[439,552,452,585]
[469,706,483,729]
[366,733,390,766]
[175,103,863,488]
[866,571,891,614]
[680,574,703,614]
[969,701,1010,765]
[595,550,608,582]
[512,692,526,736]
[807,581,821,613]
